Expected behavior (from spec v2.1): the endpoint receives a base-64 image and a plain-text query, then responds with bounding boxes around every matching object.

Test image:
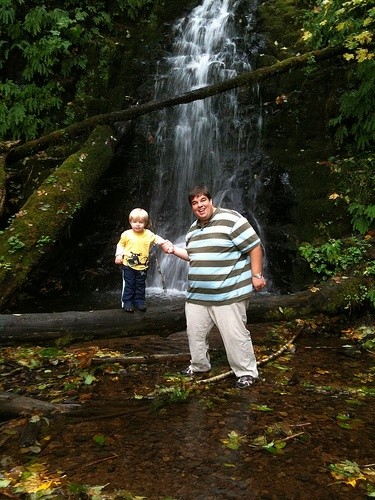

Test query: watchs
[252,274,262,279]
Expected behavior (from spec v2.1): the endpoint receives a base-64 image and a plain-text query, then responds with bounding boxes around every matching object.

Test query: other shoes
[137,305,147,311]
[236,376,256,388]
[181,367,211,375]
[124,306,134,312]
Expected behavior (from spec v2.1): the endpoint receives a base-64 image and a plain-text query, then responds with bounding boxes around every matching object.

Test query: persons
[115,208,173,312]
[161,188,266,389]
